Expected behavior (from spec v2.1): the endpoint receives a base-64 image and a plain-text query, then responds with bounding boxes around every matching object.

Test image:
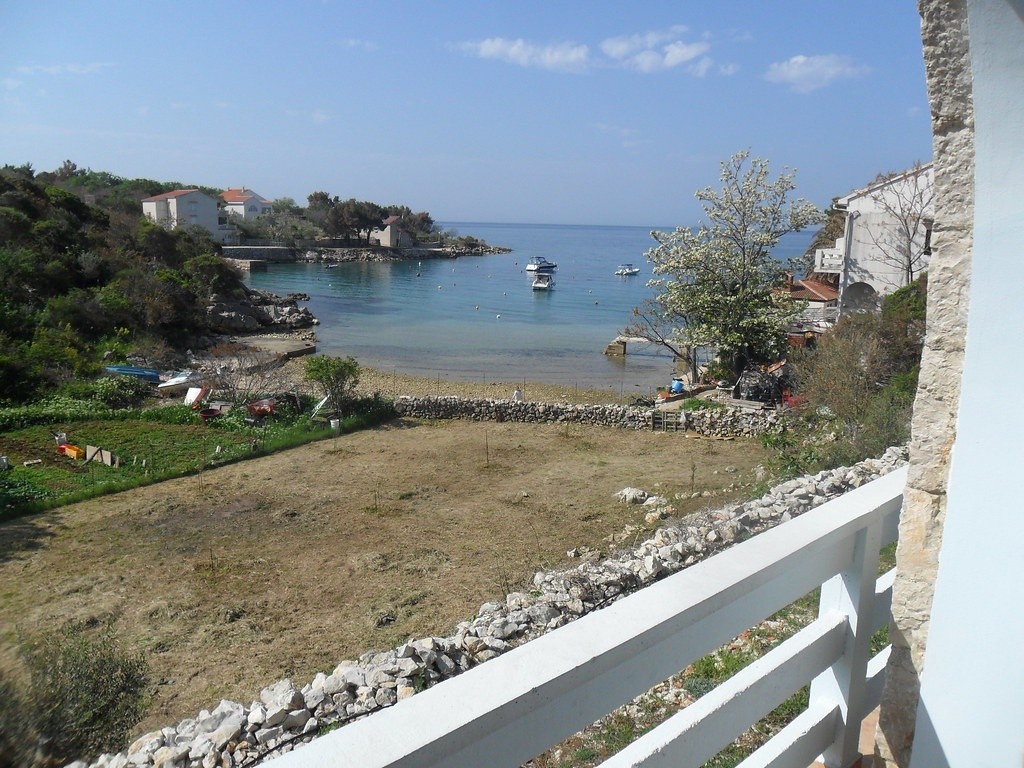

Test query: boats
[614,264,640,276]
[106,366,161,387]
[532,273,556,291]
[526,256,557,271]
[159,370,234,395]
